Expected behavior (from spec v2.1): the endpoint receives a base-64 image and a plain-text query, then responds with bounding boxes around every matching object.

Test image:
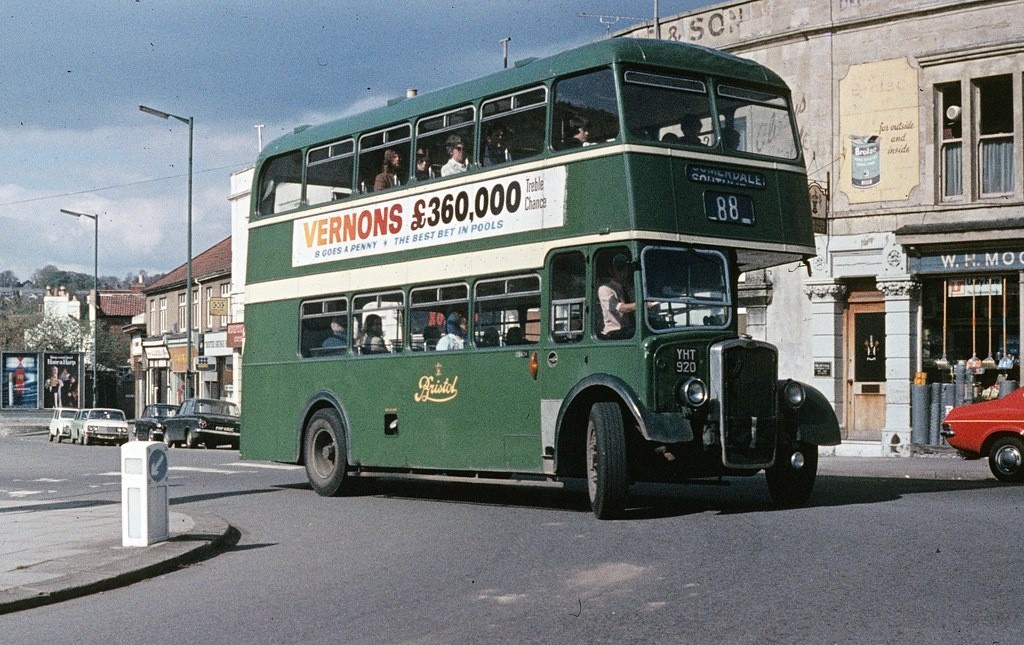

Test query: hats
[622,285,635,325]
[612,254,631,266]
[416,148,431,161]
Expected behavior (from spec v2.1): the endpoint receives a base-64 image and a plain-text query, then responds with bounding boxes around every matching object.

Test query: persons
[356,314,387,352]
[663,115,740,151]
[440,134,472,176]
[416,147,432,180]
[321,316,347,347]
[423,311,467,351]
[565,115,589,148]
[598,265,653,335]
[373,148,403,192]
[483,326,522,346]
[481,123,507,166]
[45,366,78,408]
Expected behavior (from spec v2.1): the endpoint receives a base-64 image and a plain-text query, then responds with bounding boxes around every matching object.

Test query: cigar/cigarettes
[9,373,13,406]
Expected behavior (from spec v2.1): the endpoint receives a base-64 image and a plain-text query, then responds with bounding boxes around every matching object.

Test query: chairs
[480,327,501,347]
[332,142,540,203]
[503,328,538,346]
[422,327,441,351]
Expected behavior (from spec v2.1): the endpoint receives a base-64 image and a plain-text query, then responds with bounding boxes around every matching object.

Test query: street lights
[59,208,98,407]
[137,103,193,399]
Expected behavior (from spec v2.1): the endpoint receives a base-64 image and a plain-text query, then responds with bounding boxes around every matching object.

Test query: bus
[238,37,843,522]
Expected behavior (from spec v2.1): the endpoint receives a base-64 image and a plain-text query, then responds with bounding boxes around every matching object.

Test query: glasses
[453,146,464,151]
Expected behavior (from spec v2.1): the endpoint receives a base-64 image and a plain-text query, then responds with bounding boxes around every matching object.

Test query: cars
[160,398,240,450]
[81,408,129,446]
[48,408,79,443]
[939,387,1024,484]
[133,403,179,442]
[69,409,91,445]
